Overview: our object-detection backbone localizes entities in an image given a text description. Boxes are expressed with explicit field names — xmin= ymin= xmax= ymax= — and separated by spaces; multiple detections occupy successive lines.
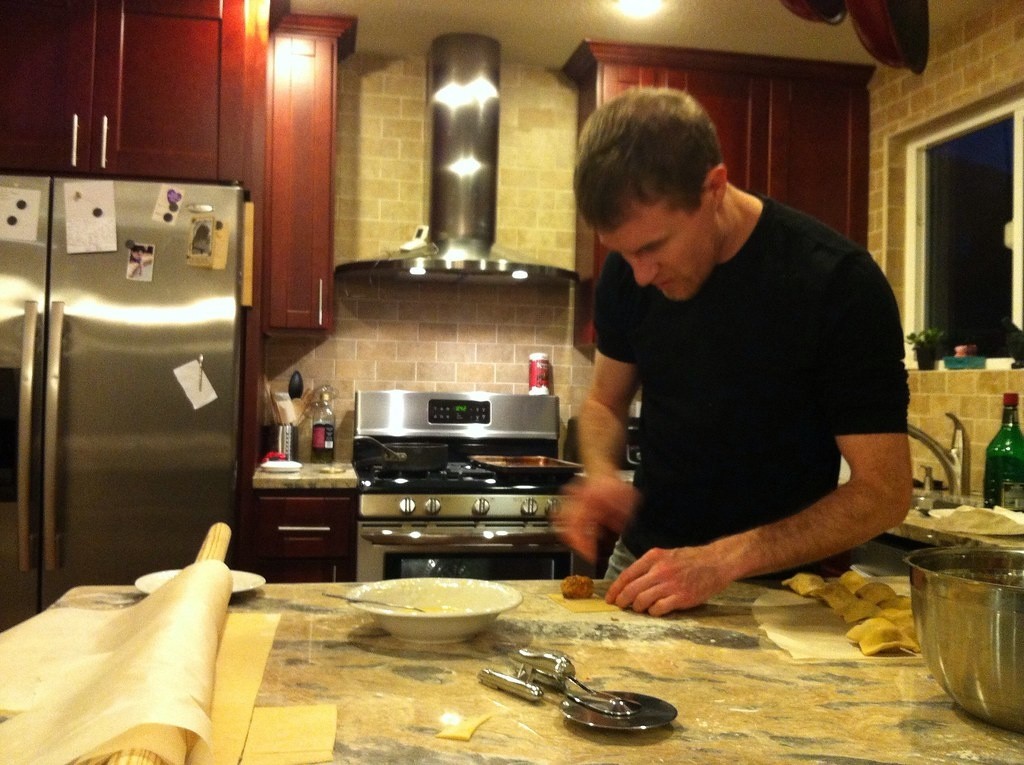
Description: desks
xmin=0 ymin=580 xmax=1024 ymax=765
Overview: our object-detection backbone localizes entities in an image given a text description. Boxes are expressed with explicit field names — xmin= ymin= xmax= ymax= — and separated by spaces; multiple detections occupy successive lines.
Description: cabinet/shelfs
xmin=262 ymin=29 xmax=337 ymax=338
xmin=560 ymin=40 xmax=877 ymax=350
xmin=251 ymin=490 xmax=359 ymax=584
xmin=0 ymin=0 xmax=271 ymax=188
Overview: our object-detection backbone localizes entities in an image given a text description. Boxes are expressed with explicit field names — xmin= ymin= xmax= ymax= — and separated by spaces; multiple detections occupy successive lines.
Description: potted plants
xmin=908 ymin=327 xmax=947 ymax=371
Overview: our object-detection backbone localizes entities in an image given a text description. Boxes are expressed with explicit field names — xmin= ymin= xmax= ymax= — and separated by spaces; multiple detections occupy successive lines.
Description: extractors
xmin=333 ymin=32 xmax=579 ymax=288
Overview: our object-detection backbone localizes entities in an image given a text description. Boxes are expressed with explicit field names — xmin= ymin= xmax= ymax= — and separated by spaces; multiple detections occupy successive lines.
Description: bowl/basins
xmin=347 ymin=577 xmax=523 ymax=644
xmin=903 ymin=546 xmax=1024 ymax=733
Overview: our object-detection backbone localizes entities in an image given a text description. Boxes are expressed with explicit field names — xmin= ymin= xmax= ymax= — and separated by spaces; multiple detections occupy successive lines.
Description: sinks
xmin=908 ymin=498 xmax=964 ymax=509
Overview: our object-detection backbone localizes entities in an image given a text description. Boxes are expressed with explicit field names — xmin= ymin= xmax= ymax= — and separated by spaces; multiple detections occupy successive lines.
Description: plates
xmin=260 ymin=460 xmax=302 ymax=472
xmin=929 ymin=507 xmax=1024 ymax=524
xmin=134 ymin=569 xmax=266 ymax=593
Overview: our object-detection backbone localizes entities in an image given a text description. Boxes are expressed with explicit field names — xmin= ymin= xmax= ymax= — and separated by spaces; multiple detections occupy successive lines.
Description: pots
xmin=353 ymin=435 xmax=448 ymax=471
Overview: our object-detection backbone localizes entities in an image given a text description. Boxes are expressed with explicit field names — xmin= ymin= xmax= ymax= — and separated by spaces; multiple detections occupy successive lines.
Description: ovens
xmin=356 ymin=521 xmax=596 ymax=583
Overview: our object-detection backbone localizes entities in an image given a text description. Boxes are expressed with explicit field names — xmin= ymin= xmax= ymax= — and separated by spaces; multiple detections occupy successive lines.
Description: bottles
xmin=311 ymin=392 xmax=335 ymax=463
xmin=982 ymin=392 xmax=1024 ymax=511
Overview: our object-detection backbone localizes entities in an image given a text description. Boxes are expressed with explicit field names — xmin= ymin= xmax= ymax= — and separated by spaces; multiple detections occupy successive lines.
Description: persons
xmin=558 ymin=88 xmax=912 ymax=616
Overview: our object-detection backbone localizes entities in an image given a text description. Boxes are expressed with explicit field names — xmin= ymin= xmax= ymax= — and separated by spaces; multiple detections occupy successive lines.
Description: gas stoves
xmin=356 ymin=390 xmax=587 ymax=523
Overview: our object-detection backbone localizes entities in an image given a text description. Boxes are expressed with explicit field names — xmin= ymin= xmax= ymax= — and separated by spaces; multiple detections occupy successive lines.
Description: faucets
xmin=907 ymin=411 xmax=971 ymax=494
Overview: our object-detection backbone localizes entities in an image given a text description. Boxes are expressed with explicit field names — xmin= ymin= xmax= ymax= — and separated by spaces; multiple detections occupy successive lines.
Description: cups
xmin=529 ymin=353 xmax=550 ymax=395
xmin=268 ymin=424 xmax=297 ymax=461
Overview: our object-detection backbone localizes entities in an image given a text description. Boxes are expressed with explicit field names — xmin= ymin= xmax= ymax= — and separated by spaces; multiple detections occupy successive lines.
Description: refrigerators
xmin=1 ymin=167 xmax=247 ymax=633
